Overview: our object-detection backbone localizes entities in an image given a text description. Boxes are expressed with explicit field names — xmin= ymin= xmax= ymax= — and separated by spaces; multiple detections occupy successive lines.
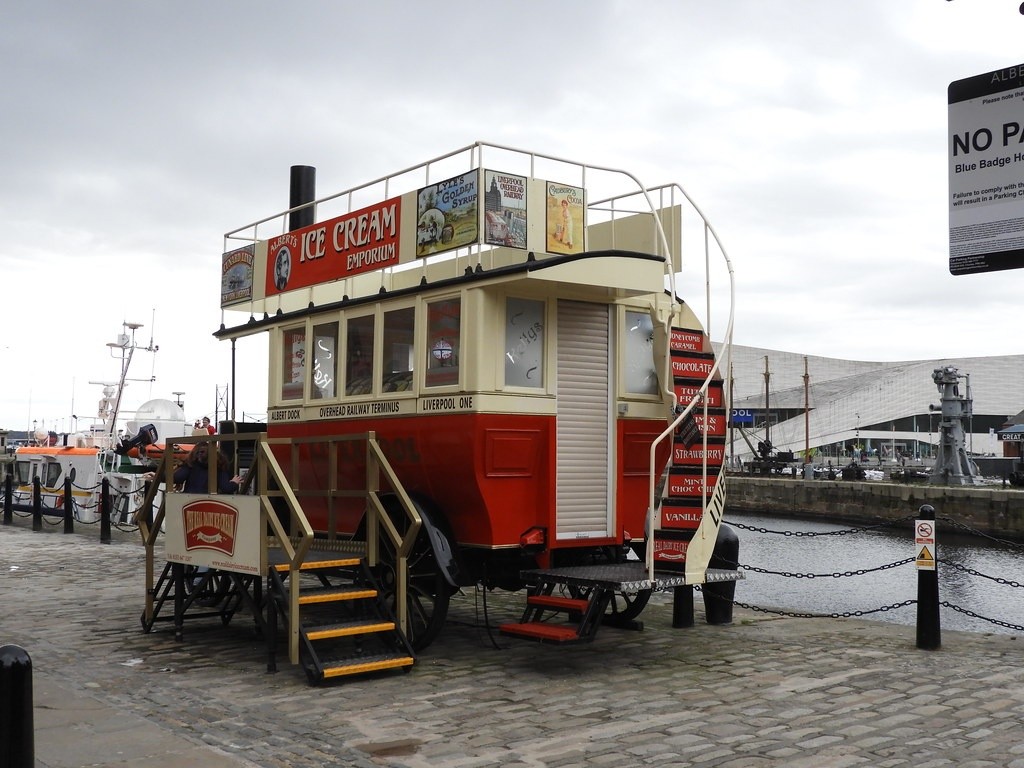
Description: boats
xmin=14 ymin=439 xmax=170 ymax=527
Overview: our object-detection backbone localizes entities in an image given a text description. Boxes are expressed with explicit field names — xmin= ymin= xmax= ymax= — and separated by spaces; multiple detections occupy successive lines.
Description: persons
xmin=142 ymin=443 xmax=244 ymax=494
xmin=201 ymin=417 xmax=215 ymax=435
